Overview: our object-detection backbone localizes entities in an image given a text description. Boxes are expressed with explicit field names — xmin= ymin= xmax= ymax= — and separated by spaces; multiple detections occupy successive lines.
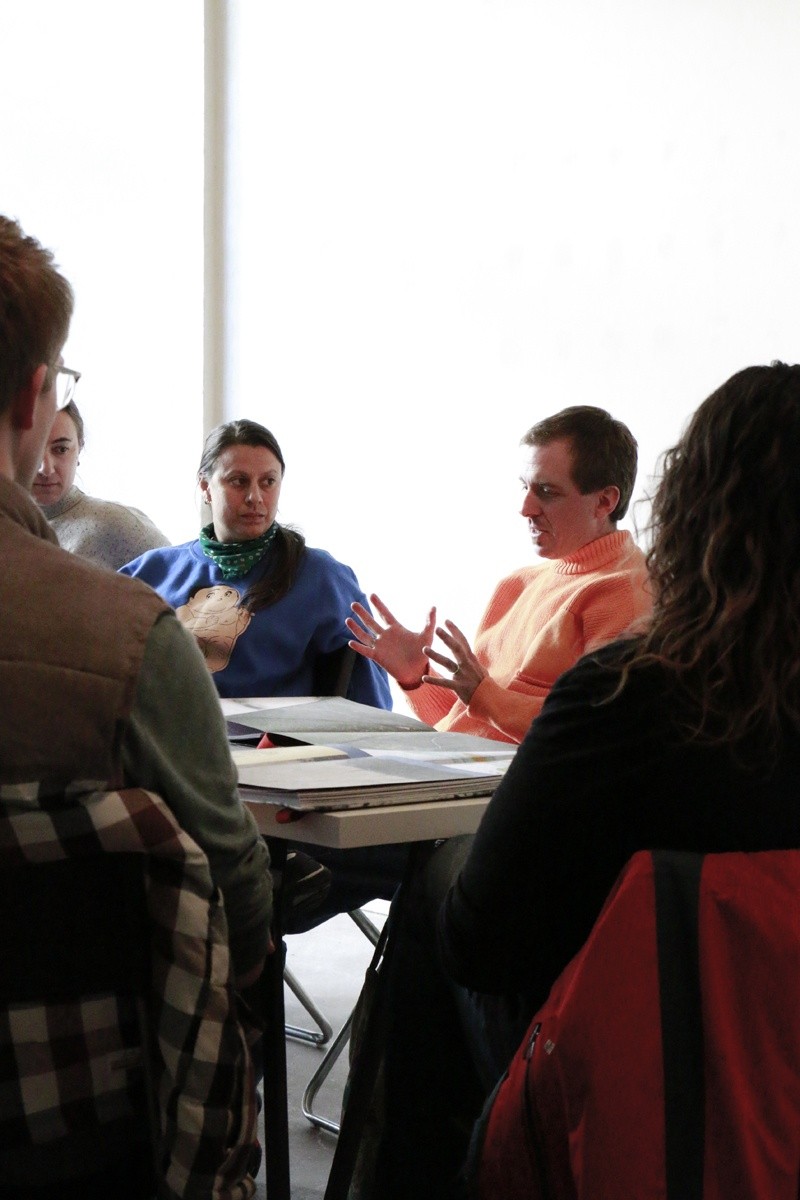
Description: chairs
xmin=468 ymin=848 xmax=800 ymax=1200
xmin=0 ymin=770 xmax=290 ymax=1200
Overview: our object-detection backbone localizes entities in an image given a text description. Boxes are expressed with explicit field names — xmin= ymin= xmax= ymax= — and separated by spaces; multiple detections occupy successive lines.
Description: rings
xmin=370 ymin=638 xmax=376 ymax=648
xmin=452 ymin=665 xmax=459 ymax=675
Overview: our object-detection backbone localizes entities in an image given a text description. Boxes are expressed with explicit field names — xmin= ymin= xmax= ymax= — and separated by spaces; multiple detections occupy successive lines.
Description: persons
xmin=0 ymin=213 xmax=387 ymax=1200
xmin=347 ymin=406 xmax=660 ymax=746
xmin=348 ymin=367 xmax=800 ymax=1200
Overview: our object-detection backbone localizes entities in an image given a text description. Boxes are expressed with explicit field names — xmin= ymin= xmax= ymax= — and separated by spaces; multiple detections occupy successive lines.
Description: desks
xmin=219 ymin=696 xmax=490 ymax=849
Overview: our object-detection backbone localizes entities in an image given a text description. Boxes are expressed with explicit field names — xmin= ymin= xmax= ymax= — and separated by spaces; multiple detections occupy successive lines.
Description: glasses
xmin=50 ymin=362 xmax=80 ymax=411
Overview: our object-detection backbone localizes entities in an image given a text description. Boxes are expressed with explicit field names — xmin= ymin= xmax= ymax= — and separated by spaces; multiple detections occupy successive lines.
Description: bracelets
xmin=398 ymin=662 xmax=430 ymax=690
